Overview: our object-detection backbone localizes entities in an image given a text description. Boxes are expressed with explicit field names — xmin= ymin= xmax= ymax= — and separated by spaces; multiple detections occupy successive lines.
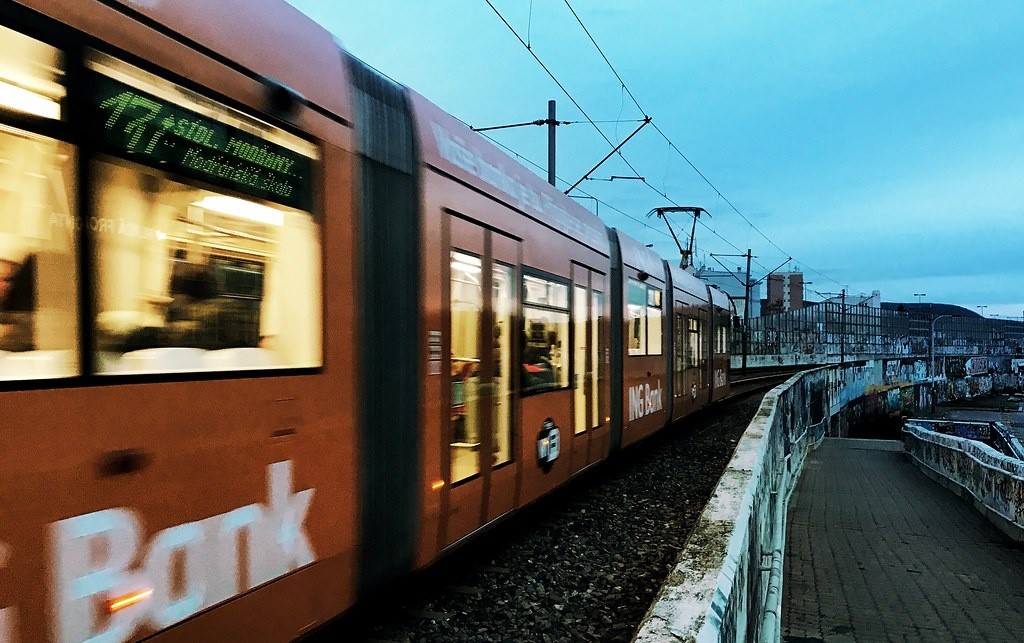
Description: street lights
xmin=930 ymin=315 xmax=953 ymax=388
xmin=914 ymin=293 xmax=926 ymax=305
xmin=977 ymin=306 xmax=987 ymax=317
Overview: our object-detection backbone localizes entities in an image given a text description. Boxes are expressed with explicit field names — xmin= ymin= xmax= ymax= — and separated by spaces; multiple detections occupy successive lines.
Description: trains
xmin=2 ymin=0 xmax=743 ymax=643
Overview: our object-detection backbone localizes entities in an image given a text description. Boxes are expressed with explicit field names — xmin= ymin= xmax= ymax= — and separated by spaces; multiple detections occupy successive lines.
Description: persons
xmin=0 ymin=259 xmax=34 ymax=351
xmin=97 ymin=264 xmax=259 ymax=351
